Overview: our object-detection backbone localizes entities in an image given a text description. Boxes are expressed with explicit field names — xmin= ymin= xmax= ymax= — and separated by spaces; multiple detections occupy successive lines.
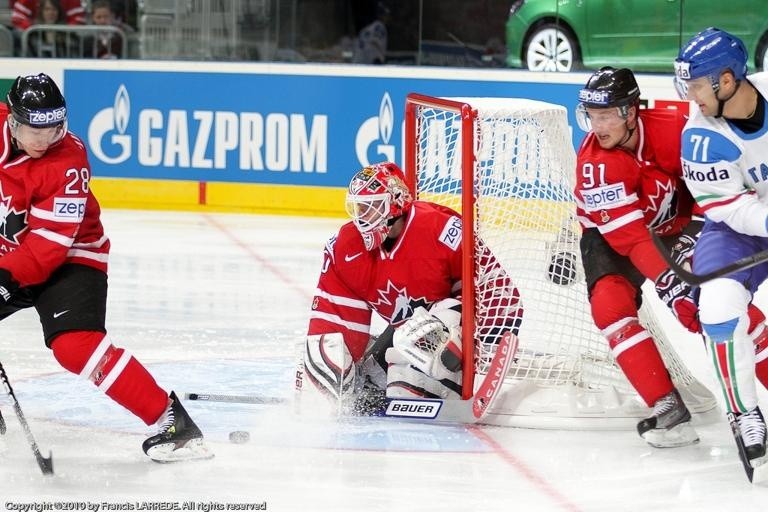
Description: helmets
xmin=345 ymin=163 xmax=414 ymax=251
xmin=576 ymin=67 xmax=640 ymax=132
xmin=673 ymin=27 xmax=748 ymax=100
xmin=7 ymin=73 xmax=67 ymax=149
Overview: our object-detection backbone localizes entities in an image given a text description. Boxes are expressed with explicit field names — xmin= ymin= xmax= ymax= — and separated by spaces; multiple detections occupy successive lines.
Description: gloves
xmin=655 ymin=269 xmax=703 ymax=334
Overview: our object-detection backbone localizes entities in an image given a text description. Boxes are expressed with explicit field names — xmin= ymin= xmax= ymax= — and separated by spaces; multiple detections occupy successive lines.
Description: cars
xmin=326 ymin=1 xmax=508 ymax=68
xmin=503 ymin=0 xmax=767 ymax=86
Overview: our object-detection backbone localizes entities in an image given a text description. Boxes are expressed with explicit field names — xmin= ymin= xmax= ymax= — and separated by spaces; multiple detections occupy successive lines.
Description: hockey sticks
xmin=649 ymin=226 xmax=766 ymax=286
xmin=183 ymin=331 xmax=519 ymax=422
xmin=1 ymin=362 xmax=54 ymax=475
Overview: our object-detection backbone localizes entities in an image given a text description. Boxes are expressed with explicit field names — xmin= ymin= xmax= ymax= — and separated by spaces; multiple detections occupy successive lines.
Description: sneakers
xmin=142 ymin=391 xmax=203 ymax=453
xmin=736 ymin=406 xmax=767 ymax=460
xmin=638 ymin=388 xmax=691 ymax=435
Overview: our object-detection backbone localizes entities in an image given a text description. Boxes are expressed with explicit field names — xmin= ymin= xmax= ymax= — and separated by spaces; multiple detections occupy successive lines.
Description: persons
xmin=574 ymin=66 xmax=767 ymax=441
xmin=1 ymin=72 xmax=215 ymax=456
xmin=304 ymin=162 xmax=523 ymax=417
xmin=0 ymin=0 xmax=514 ymax=66
xmin=673 ymin=27 xmax=767 ymax=482
